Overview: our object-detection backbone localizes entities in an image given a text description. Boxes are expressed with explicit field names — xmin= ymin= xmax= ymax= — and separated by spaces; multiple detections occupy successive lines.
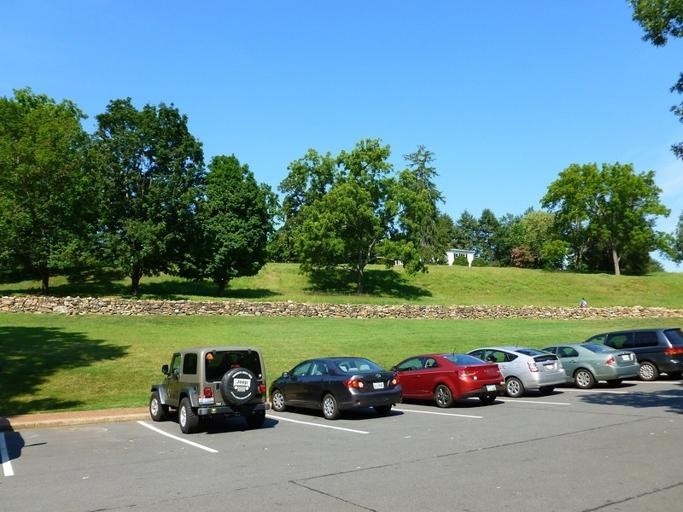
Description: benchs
xmin=340 ymin=365 xmax=370 ymax=373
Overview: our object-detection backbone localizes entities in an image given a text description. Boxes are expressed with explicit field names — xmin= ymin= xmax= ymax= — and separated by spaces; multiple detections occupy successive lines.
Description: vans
xmin=583 ymin=327 xmax=683 ymax=381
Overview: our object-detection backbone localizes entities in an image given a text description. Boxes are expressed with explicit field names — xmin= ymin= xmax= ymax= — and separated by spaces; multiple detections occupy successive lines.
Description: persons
xmin=580 ymin=297 xmax=588 ymax=307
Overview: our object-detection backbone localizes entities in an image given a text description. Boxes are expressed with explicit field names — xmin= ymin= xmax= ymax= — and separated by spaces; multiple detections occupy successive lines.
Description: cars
xmin=465 ymin=345 xmax=567 ymax=398
xmin=268 ymin=357 xmax=403 ymax=420
xmin=388 ymin=348 xmax=506 ymax=408
xmin=542 ymin=342 xmax=641 ymax=388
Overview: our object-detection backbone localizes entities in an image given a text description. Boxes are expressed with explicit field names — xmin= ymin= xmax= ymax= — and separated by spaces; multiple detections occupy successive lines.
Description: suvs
xmin=148 ymin=345 xmax=270 ymax=433
